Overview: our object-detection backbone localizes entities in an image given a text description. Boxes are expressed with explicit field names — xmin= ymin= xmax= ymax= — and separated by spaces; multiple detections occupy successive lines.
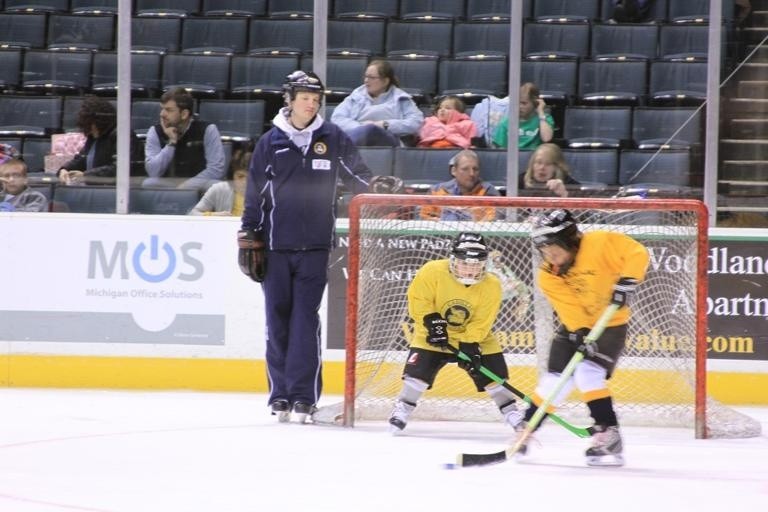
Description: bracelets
xmin=168 ymin=141 xmax=177 ymax=147
xmin=539 ymin=115 xmax=546 ymax=121
xmin=382 ymin=120 xmax=390 ymax=131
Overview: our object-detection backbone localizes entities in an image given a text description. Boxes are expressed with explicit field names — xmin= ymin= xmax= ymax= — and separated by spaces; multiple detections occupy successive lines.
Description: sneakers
xmin=272 ymin=401 xmax=292 ymax=411
xmin=295 ymin=401 xmax=317 ymax=414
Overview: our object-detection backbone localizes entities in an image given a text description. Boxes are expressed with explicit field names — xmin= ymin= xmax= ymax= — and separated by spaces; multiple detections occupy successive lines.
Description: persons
xmin=0 ymin=159 xmax=50 ymax=211
xmin=143 ymin=88 xmax=226 ymax=192
xmin=418 ymin=96 xmax=476 ymax=148
xmin=388 ymin=233 xmax=522 ymax=431
xmin=518 ymin=144 xmax=587 ymax=198
xmin=329 ymin=59 xmax=425 ymax=147
xmin=54 ymin=97 xmax=143 ymax=183
xmin=186 ymin=149 xmax=251 ymax=217
xmin=522 ymin=209 xmax=652 ymax=456
xmin=237 ymin=70 xmax=405 ymax=415
xmin=412 ymin=149 xmax=506 ymax=221
xmin=490 ymin=82 xmax=555 ymax=149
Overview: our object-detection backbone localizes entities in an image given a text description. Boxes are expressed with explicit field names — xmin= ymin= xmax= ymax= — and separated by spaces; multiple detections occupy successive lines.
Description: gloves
xmin=238 ymin=227 xmax=268 ymax=283
xmin=424 ymin=312 xmax=448 ymax=350
xmin=369 ymin=174 xmax=405 ymax=194
xmin=458 ymin=342 xmax=482 ymax=374
xmin=569 ymin=327 xmax=599 ymax=359
xmin=612 ymin=277 xmax=638 ymax=305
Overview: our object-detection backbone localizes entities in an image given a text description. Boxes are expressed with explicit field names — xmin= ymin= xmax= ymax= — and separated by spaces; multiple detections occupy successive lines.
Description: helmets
xmin=282 ymin=70 xmax=325 ymax=99
xmin=448 ymin=232 xmax=488 ymax=285
xmin=531 ymin=209 xmax=578 ymax=266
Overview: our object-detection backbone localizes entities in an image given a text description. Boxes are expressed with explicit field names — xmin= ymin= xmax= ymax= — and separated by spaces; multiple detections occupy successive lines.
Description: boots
xmin=389 ymin=398 xmax=417 ymax=430
xmin=501 ymin=399 xmax=536 ymax=453
xmin=586 ymin=425 xmax=623 ymax=456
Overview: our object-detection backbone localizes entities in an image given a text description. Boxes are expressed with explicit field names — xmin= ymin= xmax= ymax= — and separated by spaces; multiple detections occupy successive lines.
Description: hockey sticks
xmin=456 ymin=304 xmax=620 ymax=467
xmin=448 ymin=344 xmax=603 ymax=437
